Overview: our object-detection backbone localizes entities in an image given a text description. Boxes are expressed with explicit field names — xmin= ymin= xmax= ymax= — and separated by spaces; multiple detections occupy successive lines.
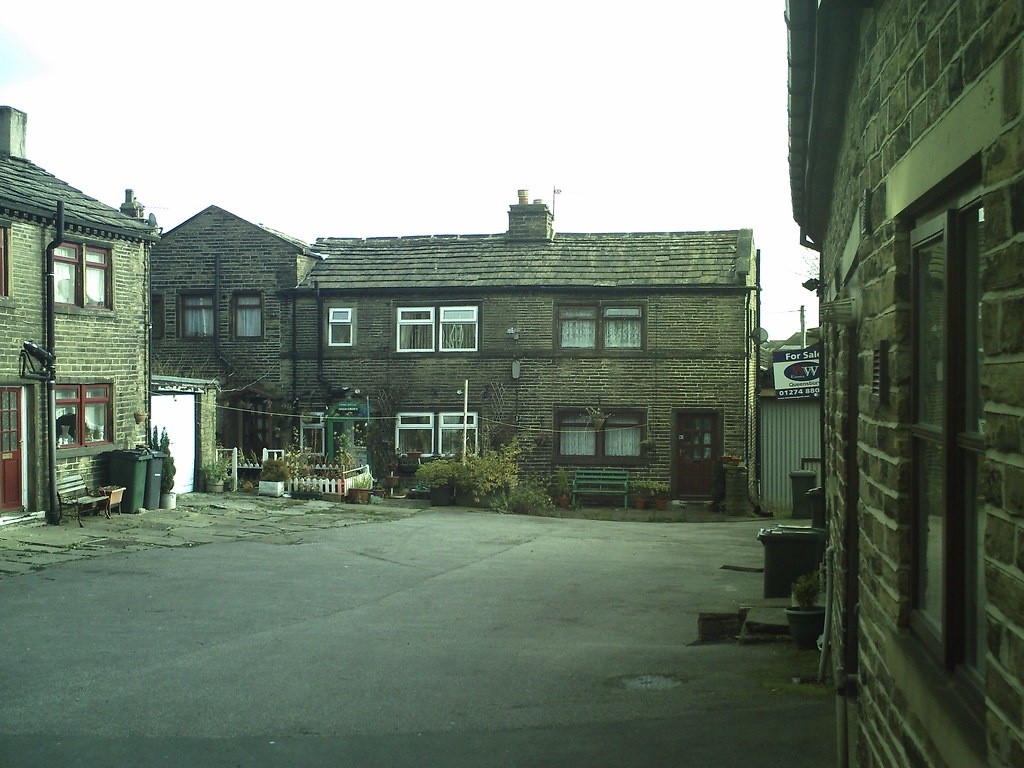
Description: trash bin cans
xmin=787 ymin=468 xmax=819 ymax=520
xmin=99 ymin=444 xmax=169 ymax=514
xmin=756 ymin=523 xmax=827 ymax=600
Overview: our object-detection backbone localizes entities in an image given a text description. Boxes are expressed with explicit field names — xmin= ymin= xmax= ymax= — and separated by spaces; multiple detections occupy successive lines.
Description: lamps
xmin=20 ymin=340 xmax=58 ymax=382
xmin=507 ymin=327 xmax=521 ymax=340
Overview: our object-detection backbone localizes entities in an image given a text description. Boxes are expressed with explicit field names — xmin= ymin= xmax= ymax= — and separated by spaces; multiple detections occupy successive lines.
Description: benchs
xmin=572 ymin=469 xmax=630 ymax=511
xmin=56 ymin=475 xmax=111 ymax=527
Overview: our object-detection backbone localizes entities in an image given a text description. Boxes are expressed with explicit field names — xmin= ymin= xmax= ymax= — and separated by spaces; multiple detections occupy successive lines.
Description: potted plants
xmin=732 ymin=455 xmax=741 ymax=466
xmin=555 ymin=467 xmax=571 ymax=509
xmin=257 ymin=457 xmax=291 ymax=497
xmin=415 ymin=448 xmax=520 ymax=508
xmin=160 ymin=427 xmax=176 ymax=510
xmin=631 ymin=478 xmax=671 ymax=511
xmin=199 ymin=457 xmax=231 ymax=492
xmin=785 ymin=569 xmax=829 ymax=653
xmin=348 ymin=460 xmax=399 ymax=503
xmin=720 ymin=453 xmax=733 ymax=464
xmin=578 ymin=405 xmax=612 ymax=430
xmin=94 ymin=480 xmax=126 ymax=519
